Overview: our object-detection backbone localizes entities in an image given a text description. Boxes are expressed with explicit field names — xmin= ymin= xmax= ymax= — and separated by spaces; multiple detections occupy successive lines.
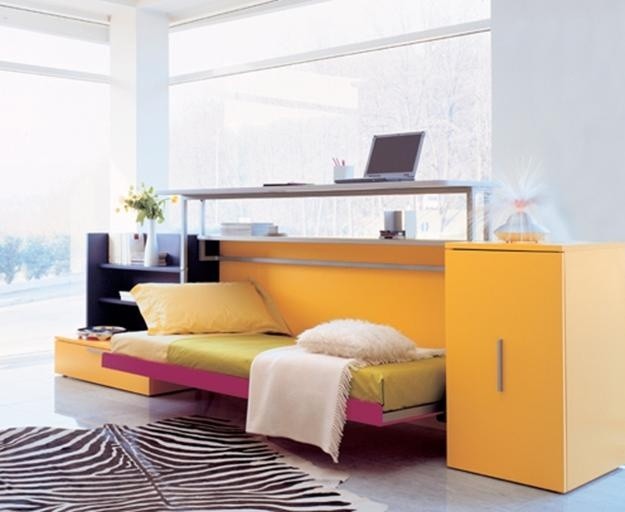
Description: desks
xmin=158 ymin=177 xmax=501 ymax=284
xmin=55 ymin=333 xmax=193 ymax=397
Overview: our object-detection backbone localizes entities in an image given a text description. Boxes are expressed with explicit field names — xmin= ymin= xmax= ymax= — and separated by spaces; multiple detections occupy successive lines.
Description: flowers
xmin=115 ymin=180 xmax=180 ymax=227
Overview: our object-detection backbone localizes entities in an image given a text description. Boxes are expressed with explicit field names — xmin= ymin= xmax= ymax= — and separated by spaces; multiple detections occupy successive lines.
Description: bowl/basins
xmin=118 ymin=291 xmax=135 ymax=302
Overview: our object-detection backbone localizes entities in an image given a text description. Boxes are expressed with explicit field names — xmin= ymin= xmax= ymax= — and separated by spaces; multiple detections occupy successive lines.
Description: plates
xmin=219 ymin=220 xmax=277 ymax=238
xmin=78 ymin=327 xmax=126 ymax=339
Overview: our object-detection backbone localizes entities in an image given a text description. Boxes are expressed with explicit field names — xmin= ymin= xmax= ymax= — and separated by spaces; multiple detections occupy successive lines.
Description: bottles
xmin=130 ymin=233 xmax=143 ymax=264
xmin=493 ymin=212 xmax=548 ymax=243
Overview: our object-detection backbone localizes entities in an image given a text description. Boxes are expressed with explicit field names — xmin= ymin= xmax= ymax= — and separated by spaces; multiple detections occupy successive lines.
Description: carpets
xmin=0 ymin=413 xmax=389 ymax=512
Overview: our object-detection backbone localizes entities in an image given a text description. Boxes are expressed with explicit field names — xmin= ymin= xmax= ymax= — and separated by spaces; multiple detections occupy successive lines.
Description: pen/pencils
xmin=333 ymin=158 xmax=346 ymax=167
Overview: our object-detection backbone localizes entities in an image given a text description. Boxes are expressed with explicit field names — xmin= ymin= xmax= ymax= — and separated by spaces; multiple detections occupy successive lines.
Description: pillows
xmin=131 ymin=280 xmax=292 ymax=336
xmin=294 ymin=317 xmax=417 ymax=364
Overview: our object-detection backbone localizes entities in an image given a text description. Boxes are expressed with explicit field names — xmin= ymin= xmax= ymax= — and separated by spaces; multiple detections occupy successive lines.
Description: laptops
xmin=334 ymin=131 xmax=425 ymax=182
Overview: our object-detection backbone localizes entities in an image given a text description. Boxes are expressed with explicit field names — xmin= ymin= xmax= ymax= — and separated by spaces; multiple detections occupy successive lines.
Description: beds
xmin=101 ymin=331 xmax=445 ymax=427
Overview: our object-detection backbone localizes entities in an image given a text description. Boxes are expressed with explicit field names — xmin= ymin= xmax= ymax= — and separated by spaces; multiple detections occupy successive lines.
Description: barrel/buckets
xmin=77 ymin=321 xmax=128 ymax=345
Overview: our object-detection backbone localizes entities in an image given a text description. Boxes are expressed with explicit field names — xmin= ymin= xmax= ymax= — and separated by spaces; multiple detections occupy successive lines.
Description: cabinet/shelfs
xmin=99 ymin=262 xmax=180 ymax=308
xmin=444 ymin=242 xmax=625 ymax=495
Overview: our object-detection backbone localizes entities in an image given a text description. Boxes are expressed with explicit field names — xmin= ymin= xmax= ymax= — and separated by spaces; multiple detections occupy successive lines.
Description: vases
xmin=143 ymin=220 xmax=159 ymax=267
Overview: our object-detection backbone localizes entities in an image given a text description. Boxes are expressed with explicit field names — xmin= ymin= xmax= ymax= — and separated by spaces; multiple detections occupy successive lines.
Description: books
xmin=221 ymin=222 xmax=279 ymax=237
xmin=108 ymin=232 xmax=168 ymax=266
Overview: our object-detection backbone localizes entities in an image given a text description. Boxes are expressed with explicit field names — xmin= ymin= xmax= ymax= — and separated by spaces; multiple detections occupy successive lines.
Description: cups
xmin=332 ymin=166 xmax=353 ymax=183
